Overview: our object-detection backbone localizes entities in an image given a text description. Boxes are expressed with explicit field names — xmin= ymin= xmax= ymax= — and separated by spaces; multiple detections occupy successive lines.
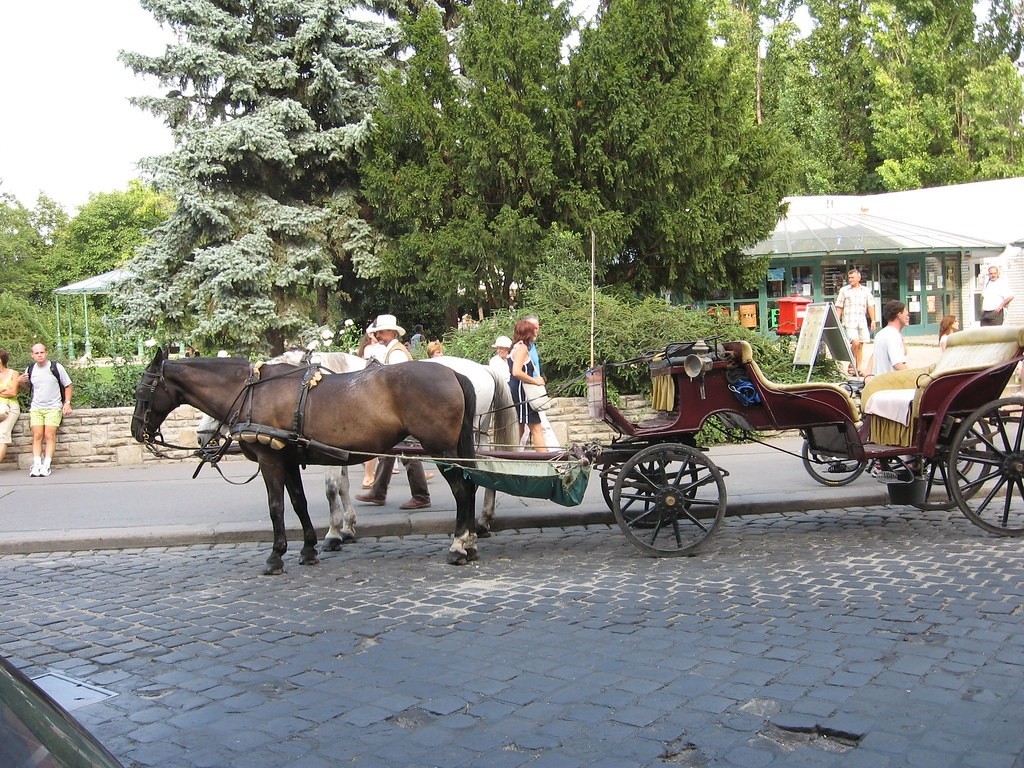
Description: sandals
xmin=858 ymin=370 xmax=865 ymax=378
xmin=849 ymin=363 xmax=855 ymax=375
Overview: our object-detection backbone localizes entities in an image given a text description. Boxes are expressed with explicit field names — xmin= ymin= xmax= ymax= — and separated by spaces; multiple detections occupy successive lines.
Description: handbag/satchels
xmin=0 ymin=402 xmax=10 ymax=421
xmin=513 ymin=344 xmax=551 ymax=411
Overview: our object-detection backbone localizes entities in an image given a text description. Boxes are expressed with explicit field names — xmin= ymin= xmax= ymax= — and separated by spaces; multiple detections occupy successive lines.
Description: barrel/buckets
xmin=887 ymin=467 xmax=927 ymax=505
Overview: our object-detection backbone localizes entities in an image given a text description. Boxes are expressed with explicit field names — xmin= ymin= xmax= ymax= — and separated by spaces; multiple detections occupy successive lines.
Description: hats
xmin=491 ymin=336 xmax=513 ymax=349
xmin=368 ymin=314 xmax=406 ymax=337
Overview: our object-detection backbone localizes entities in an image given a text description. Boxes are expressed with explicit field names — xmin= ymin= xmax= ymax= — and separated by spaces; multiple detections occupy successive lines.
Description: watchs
xmin=64 ymin=400 xmax=71 ymax=404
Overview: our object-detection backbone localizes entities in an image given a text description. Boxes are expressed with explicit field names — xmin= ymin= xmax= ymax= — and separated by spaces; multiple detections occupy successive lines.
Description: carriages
xmin=130 ymin=325 xmax=1023 ymax=576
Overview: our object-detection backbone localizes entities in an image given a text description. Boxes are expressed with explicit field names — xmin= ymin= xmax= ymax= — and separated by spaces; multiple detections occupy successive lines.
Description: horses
xmin=196 ymin=350 xmax=520 ymax=551
xmin=131 ymin=347 xmax=480 ymax=576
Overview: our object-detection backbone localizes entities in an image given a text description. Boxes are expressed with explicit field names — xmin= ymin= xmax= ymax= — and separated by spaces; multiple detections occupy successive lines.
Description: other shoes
xmin=362 ymin=482 xmax=375 ymax=490
xmin=391 ymin=469 xmax=400 ymax=474
xmin=425 ymin=472 xmax=434 ymax=479
xmin=872 ymin=467 xmax=883 ymax=477
xmin=399 ymin=495 xmax=431 ymax=510
xmin=355 ymin=491 xmax=386 ymax=505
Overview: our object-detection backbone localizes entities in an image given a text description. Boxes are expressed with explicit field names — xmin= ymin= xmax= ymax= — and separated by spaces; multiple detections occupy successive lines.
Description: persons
xmin=0 ymin=350 xmax=20 ymax=462
xmin=980 ymin=266 xmax=1013 ymax=325
xmin=520 ymin=316 xmax=561 ymax=451
xmin=865 ymin=300 xmax=909 ymax=384
xmin=488 ymin=336 xmax=511 ymax=449
xmin=938 ymin=314 xmax=959 ymax=351
xmin=18 ymin=344 xmax=73 ymax=477
xmin=355 ymin=314 xmax=435 ymax=509
xmin=835 ymin=268 xmax=876 ymax=377
xmin=507 ymin=321 xmax=547 ymax=453
xmin=427 ymin=341 xmax=443 ymax=358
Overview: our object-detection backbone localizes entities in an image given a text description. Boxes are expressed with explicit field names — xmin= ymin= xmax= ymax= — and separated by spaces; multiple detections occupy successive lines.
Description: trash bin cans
xmin=775 ymin=297 xmax=813 ymax=336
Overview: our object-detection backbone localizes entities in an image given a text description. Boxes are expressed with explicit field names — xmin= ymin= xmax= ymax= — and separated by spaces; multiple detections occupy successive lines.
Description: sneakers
xmin=30 ymin=464 xmax=41 ymax=477
xmin=41 ymin=462 xmax=51 ymax=478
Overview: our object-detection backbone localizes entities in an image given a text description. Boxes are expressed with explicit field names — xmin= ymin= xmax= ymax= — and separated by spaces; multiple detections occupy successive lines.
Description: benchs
xmin=645 ymin=335 xmax=727 ymax=377
xmin=723 ymin=341 xmax=860 ymax=422
xmin=860 ymin=325 xmax=1024 ymax=413
